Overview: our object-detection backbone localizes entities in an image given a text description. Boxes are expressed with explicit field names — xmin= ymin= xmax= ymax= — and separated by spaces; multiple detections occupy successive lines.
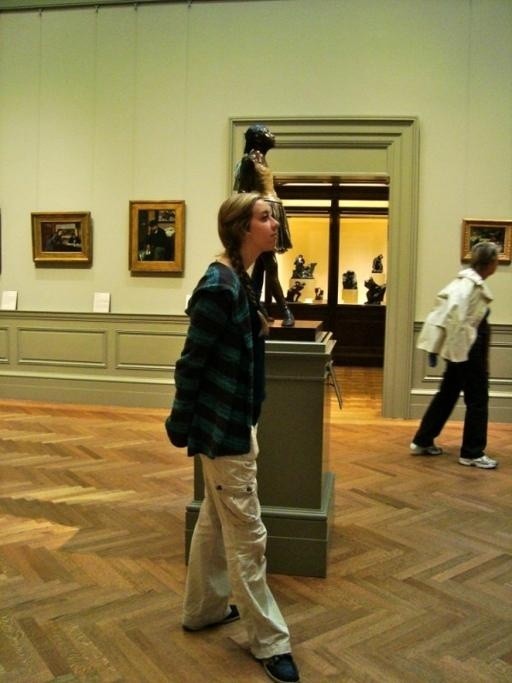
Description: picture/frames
xmin=30 ymin=210 xmax=90 ymax=265
xmin=127 ymin=198 xmax=186 ymax=276
xmin=461 ymin=218 xmax=512 ymax=266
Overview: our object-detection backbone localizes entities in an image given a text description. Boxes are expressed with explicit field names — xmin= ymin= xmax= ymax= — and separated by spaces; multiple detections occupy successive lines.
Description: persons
xmin=408 ymin=240 xmax=499 ymax=469
xmin=232 ymin=124 xmax=294 ymax=328
xmin=45 ymin=229 xmax=65 ymax=252
xmin=165 ymin=192 xmax=300 ymax=683
xmin=144 ymin=219 xmax=170 ymax=261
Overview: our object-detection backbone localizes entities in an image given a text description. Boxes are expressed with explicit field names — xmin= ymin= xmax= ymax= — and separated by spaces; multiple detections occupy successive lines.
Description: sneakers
xmin=183 ymin=604 xmax=239 ymax=632
xmin=261 ymin=654 xmax=300 ymax=683
xmin=458 ymin=455 xmax=498 ymax=469
xmin=409 ymin=442 xmax=442 ymax=455
